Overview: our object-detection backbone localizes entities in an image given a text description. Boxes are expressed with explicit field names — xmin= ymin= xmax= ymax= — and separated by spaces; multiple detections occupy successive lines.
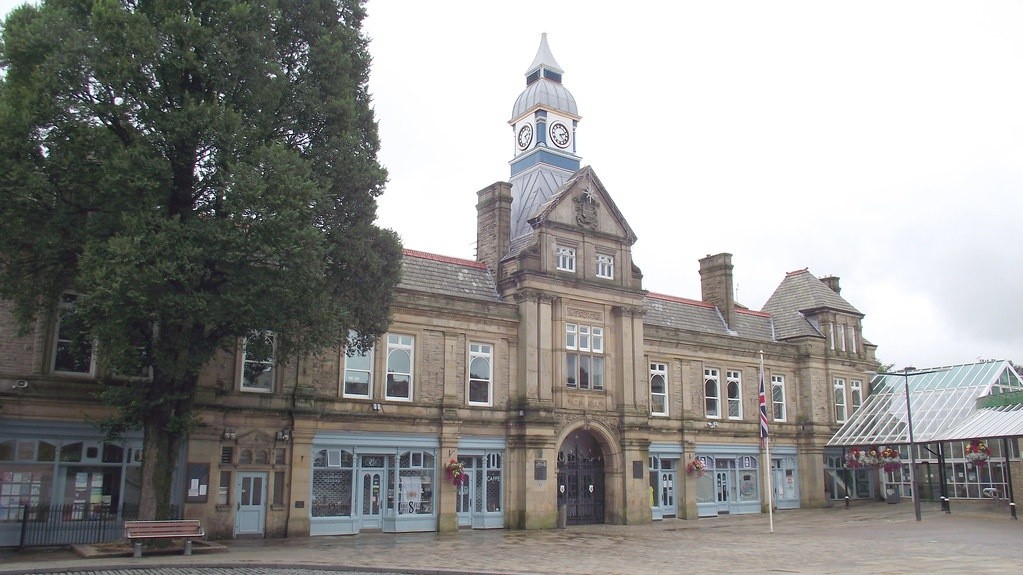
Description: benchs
xmin=123 ymin=520 xmax=206 ymax=557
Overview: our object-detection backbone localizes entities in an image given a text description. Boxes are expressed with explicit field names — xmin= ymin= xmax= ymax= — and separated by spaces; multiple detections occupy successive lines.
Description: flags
xmin=758 ymin=378 xmax=768 ymax=441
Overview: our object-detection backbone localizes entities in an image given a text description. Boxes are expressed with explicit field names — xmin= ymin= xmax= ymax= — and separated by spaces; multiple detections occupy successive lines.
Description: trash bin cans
xmin=886 ymin=483 xmax=900 ymax=504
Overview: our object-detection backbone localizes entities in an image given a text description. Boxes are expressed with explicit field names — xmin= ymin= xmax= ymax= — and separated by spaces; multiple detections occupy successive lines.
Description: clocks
xmin=550 ymin=120 xmax=572 ymax=148
xmin=517 ymin=123 xmax=533 ymax=150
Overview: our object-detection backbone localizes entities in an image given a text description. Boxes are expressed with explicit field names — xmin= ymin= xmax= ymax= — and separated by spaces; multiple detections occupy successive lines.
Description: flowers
xmin=446 ymin=459 xmax=466 ymax=487
xmin=966 ymin=437 xmax=993 ymax=465
xmin=844 ymin=447 xmax=902 ymax=471
xmin=687 ymin=458 xmax=705 ymax=478
